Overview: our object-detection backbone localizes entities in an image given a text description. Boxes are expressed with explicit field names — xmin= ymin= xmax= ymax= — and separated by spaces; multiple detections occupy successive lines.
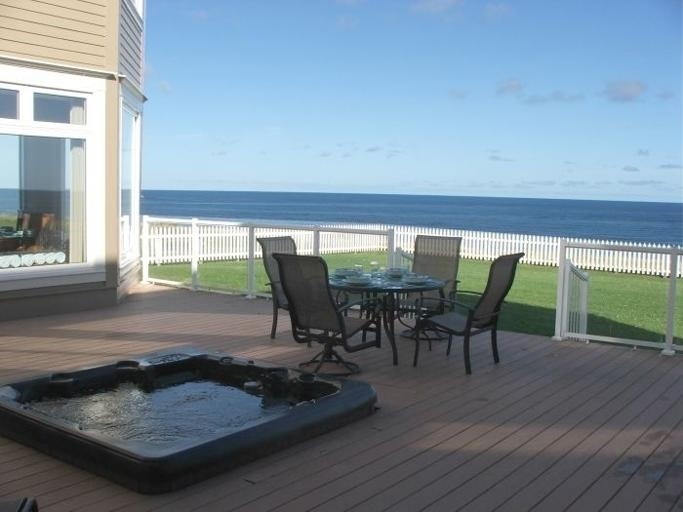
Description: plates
xmin=401 ymin=279 xmax=432 ymax=285
xmin=342 ymin=279 xmax=371 ymax=286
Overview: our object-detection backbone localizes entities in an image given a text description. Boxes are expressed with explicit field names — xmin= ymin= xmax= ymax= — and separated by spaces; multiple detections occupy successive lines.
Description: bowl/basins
xmin=346 ymin=275 xmax=369 ymax=282
xmin=407 ymin=275 xmax=428 ymax=281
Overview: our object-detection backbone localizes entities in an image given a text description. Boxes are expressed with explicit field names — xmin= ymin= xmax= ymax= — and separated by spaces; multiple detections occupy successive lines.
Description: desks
xmin=329 ymin=269 xmax=447 ymax=366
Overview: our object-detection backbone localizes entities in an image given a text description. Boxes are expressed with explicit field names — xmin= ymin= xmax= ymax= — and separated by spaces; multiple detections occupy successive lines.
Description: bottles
xmin=355 ymin=263 xmax=362 ymax=276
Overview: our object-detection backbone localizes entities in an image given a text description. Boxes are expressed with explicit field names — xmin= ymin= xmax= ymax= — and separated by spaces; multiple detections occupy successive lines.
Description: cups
xmin=369 ymin=261 xmax=383 ymax=279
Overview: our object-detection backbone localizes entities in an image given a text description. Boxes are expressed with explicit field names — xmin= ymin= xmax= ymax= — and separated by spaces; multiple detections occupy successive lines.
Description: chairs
xmin=256 ymin=235 xmax=342 ymax=345
xmin=272 ymin=252 xmax=373 ymax=377
xmin=413 ymin=252 xmax=526 ymax=374
xmin=395 ymin=234 xmax=462 ymax=350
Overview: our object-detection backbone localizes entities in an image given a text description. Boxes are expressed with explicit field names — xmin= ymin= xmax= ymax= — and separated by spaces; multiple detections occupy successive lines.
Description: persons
xmin=14 ymin=212 xmax=53 ymax=252
